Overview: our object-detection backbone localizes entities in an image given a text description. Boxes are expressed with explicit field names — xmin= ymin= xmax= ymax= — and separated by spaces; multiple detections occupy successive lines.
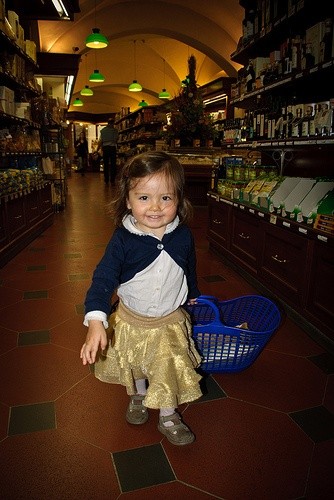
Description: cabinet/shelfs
xmin=0 ymin=0 xmax=57 ymax=269
xmin=114 ymin=106 xmax=158 ymax=144
xmin=184 ymin=1 xmax=334 ymax=372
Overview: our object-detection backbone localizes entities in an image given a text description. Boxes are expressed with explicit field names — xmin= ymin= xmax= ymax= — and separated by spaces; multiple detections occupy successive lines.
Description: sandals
xmin=127 ymin=395 xmax=148 ymax=425
xmin=157 ymin=413 xmax=195 ymax=445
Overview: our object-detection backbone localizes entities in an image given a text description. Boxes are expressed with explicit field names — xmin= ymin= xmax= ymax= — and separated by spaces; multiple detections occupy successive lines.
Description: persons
xmin=97 ymin=119 xmax=120 ymax=184
xmin=74 ymin=132 xmax=89 ymax=176
xmin=80 ymin=152 xmax=201 ymax=446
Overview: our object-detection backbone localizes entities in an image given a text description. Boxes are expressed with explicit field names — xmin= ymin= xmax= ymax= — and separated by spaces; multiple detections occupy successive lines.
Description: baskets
xmin=183 ymin=295 xmax=280 ymax=373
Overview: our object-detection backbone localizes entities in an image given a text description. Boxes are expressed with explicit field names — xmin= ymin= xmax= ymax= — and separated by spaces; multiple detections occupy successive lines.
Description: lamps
xmin=159 ymin=58 xmax=172 ymax=98
xmin=72 ymin=97 xmax=84 ymax=107
xmin=180 ymin=49 xmax=190 ymax=86
xmin=86 ymin=0 xmax=109 ymax=48
xmin=129 ymin=40 xmax=142 ymax=92
xmin=89 ymin=49 xmax=105 ymax=82
xmin=79 ymin=53 xmax=94 ymax=96
xmin=138 ymin=99 xmax=147 ymax=107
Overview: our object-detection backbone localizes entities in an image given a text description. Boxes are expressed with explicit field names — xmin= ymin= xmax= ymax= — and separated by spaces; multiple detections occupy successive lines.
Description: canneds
xmin=217 ymin=163 xmax=278 ymax=200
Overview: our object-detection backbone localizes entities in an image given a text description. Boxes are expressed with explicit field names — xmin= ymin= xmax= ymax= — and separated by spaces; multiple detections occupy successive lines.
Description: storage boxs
xmin=0 ymin=9 xmax=36 ymax=120
xmin=246 ymin=22 xmax=330 ymax=85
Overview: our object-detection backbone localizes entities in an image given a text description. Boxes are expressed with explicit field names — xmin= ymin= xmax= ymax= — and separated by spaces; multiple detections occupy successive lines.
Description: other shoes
xmin=104 ymin=179 xmax=109 ymax=183
xmin=111 ymin=179 xmax=115 ymax=182
